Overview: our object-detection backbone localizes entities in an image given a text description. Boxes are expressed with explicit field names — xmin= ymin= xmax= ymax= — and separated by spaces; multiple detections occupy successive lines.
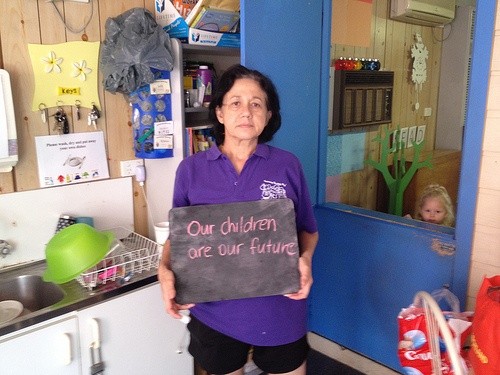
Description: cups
xmin=77 ymin=217 xmax=93 ymax=227
xmin=55 ymin=216 xmax=77 ymax=233
xmin=154 ymin=222 xmax=170 ymax=254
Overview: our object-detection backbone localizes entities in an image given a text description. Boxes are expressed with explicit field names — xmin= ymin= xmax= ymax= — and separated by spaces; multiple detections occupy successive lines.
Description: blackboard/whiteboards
xmin=167 ymin=198 xmax=300 ymax=305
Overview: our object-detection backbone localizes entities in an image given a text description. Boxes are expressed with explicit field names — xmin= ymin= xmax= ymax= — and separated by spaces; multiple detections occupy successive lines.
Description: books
xmin=173 ymin=0 xmax=240 ymax=33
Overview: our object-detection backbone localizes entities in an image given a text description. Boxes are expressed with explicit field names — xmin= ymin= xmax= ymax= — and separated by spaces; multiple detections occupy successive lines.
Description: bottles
xmin=197 ymin=65 xmax=212 ymax=102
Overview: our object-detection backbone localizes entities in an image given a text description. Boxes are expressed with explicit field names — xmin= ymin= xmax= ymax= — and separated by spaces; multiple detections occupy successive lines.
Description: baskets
xmin=75 ymin=224 xmax=166 ymax=290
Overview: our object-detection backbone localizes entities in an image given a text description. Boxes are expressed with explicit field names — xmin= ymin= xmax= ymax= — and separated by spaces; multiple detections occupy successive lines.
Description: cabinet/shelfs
xmin=144 ymin=38 xmax=241 ymax=243
xmin=0 ymin=234 xmax=195 ymax=375
xmin=392 ymin=148 xmax=460 ymax=228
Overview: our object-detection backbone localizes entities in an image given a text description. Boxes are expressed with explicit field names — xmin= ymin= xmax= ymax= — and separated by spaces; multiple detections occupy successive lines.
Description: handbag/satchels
xmin=468 ymin=276 xmax=500 ymax=375
xmin=398 ymin=286 xmax=472 ymax=374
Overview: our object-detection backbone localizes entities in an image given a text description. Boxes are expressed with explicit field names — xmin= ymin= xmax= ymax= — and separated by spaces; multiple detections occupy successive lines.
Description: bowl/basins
xmin=43 ymin=223 xmax=114 ymax=285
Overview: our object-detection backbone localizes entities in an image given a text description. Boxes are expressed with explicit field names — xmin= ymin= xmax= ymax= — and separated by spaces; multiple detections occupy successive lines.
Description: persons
xmin=158 ymin=64 xmax=316 ymax=375
xmin=402 ymin=183 xmax=455 ymax=226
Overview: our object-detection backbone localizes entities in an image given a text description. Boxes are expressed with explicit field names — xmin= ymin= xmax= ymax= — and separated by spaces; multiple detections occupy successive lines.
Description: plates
xmin=0 ymin=300 xmax=23 ymax=324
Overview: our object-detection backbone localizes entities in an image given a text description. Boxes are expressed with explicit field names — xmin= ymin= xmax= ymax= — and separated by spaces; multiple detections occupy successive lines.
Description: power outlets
xmin=120 ymin=158 xmax=144 ymax=177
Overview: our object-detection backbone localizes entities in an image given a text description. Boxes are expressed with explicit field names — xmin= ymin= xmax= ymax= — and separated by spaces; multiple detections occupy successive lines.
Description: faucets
xmin=0 ymin=240 xmax=12 ymax=258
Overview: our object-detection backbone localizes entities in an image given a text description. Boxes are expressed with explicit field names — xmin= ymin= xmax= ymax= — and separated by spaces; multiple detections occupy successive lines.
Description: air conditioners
xmin=389 ymin=0 xmax=455 ymax=28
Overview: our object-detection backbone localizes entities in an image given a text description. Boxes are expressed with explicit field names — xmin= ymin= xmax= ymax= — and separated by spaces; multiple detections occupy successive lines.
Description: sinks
xmin=0 ymin=271 xmax=68 ymax=327
